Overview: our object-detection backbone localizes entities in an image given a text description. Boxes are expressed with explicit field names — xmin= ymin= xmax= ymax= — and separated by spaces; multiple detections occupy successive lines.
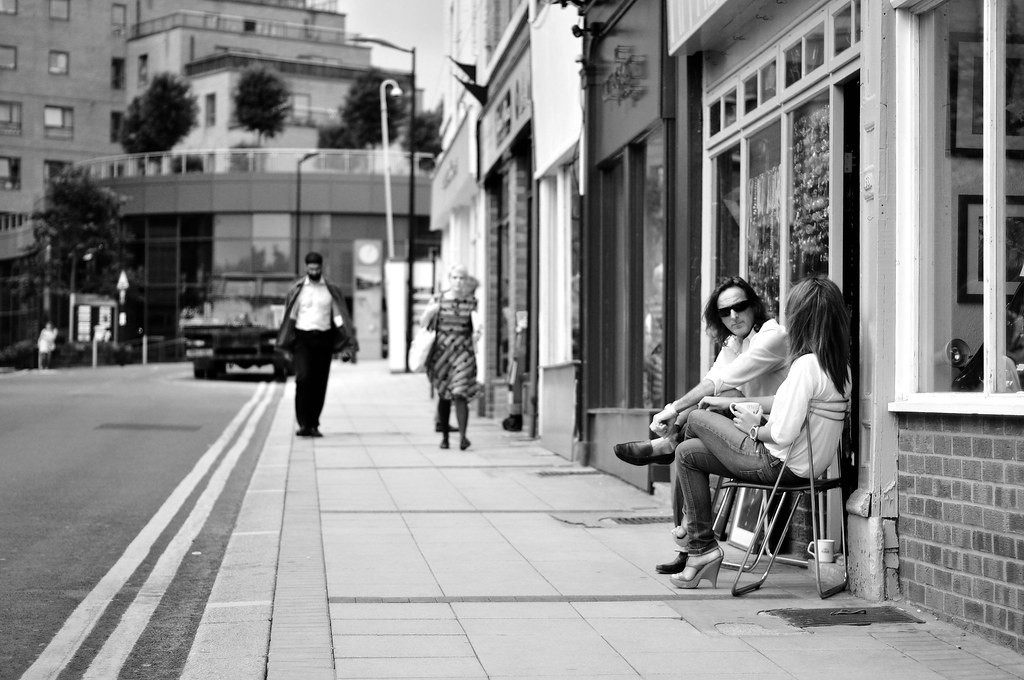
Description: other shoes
xmin=459 ymin=438 xmax=470 ymax=450
xmin=439 ymin=435 xmax=450 ymax=448
xmin=296 ymin=426 xmax=323 ymax=437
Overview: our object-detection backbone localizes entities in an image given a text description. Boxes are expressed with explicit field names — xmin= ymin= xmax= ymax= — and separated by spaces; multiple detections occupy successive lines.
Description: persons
xmin=613 ymin=277 xmax=790 ymax=573
xmin=419 ymin=263 xmax=481 ymax=450
xmin=643 ymin=264 xmax=664 ymax=410
xmin=38 ymin=320 xmax=59 ymax=370
xmin=668 ymin=277 xmax=852 ymax=589
xmin=275 ymin=251 xmax=356 ymax=437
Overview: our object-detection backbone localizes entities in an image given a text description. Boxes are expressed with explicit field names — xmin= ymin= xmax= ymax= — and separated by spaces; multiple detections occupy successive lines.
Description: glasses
xmin=713 ymin=300 xmax=749 ymax=319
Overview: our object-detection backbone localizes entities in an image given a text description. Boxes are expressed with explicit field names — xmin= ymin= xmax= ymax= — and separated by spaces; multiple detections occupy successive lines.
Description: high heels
xmin=670 ymin=526 xmax=691 ymax=546
xmin=670 ymin=551 xmax=724 ymax=590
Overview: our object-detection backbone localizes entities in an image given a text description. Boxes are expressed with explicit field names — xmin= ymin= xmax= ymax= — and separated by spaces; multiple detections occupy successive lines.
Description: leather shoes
xmin=656 ymin=551 xmax=688 ymax=574
xmin=613 ymin=440 xmax=675 ymax=467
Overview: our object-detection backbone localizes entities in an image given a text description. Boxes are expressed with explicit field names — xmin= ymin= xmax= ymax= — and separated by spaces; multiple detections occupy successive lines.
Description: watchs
xmin=749 ymin=424 xmax=762 ymax=441
xmin=665 ymin=404 xmax=679 ymax=417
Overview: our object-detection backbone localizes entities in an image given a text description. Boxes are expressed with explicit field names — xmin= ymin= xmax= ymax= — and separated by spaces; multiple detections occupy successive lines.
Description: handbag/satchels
xmin=408 ymin=295 xmax=441 ymax=371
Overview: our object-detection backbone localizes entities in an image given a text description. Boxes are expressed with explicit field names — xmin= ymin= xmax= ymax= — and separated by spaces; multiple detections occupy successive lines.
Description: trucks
xmin=178 ymin=273 xmax=301 ymax=383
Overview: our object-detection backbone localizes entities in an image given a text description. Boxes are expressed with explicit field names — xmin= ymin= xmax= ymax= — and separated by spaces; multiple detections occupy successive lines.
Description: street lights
xmin=295 ymin=151 xmax=321 ymax=274
xmin=345 ymin=37 xmax=418 ymax=374
xmin=379 ymin=78 xmax=403 ymax=359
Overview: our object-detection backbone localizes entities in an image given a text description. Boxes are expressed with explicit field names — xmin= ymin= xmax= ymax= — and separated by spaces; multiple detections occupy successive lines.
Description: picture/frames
xmin=949 ymin=33 xmax=1024 ymax=156
xmin=956 ymin=193 xmax=1024 ymax=304
xmin=726 ymin=486 xmax=772 ymax=554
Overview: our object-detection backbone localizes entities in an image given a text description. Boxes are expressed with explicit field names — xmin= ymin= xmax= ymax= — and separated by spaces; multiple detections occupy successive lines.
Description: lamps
xmin=932 ymin=338 xmax=969 ymax=371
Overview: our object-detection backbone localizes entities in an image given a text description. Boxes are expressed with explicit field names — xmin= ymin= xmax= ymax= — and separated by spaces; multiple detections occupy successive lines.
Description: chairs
xmin=720 ymin=399 xmax=851 ymax=599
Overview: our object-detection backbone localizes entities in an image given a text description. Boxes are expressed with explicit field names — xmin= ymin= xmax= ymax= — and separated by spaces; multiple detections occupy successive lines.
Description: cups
xmin=729 ymin=402 xmax=759 ymax=417
xmin=807 ymin=540 xmax=835 ymax=562
